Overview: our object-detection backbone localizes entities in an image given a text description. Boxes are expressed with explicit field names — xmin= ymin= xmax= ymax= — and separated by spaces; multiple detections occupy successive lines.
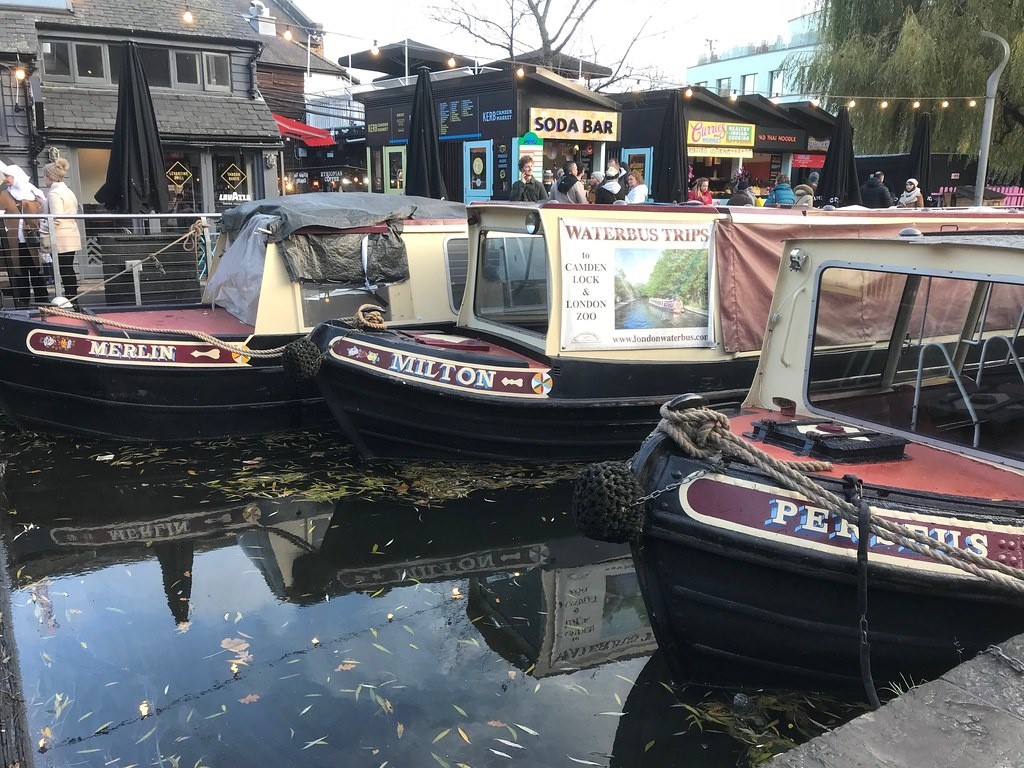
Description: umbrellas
xmin=813 ymin=106 xmax=862 ymax=208
xmin=338 ymin=39 xmax=479 ymax=86
xmin=405 ymin=66 xmax=450 ymax=201
xmin=478 ymin=47 xmax=613 ymax=79
xmin=898 ymin=112 xmax=933 ymax=207
xmin=94 ymin=37 xmax=175 ymax=234
xmin=646 ymin=90 xmax=689 ymax=203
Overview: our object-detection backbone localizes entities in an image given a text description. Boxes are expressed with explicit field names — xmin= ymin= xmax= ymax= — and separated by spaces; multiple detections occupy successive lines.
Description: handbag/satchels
xmin=27 ymin=229 xmax=40 ymax=246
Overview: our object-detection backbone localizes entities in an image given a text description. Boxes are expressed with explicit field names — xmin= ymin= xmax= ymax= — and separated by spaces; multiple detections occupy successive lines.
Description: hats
xmin=605 ymin=167 xmax=619 ymax=180
xmin=907 ymin=178 xmax=918 ymax=187
xmin=738 ymin=180 xmax=749 ymax=190
xmin=43 ymin=158 xmax=69 ymax=182
xmin=591 ymin=171 xmax=604 ymax=181
xmin=809 ymin=172 xmax=819 ymax=182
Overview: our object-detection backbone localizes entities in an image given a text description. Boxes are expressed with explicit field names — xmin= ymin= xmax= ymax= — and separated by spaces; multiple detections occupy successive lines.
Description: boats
xmin=648 ymin=296 xmax=685 ymax=315
xmin=0 ymin=191 xmax=554 ymax=452
xmin=0 ymin=420 xmax=657 ymax=682
xmin=568 ymin=229 xmax=1024 ymax=704
xmin=281 ymin=200 xmax=1024 ymax=463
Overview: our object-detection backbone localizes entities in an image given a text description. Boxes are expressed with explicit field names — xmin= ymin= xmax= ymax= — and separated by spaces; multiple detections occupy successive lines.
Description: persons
xmin=687 ymin=172 xmax=820 ymax=209
xmin=0 ymin=157 xmax=83 ymax=310
xmin=856 ymin=171 xmax=924 ymax=208
xmin=544 ymin=157 xmax=648 ymax=204
xmin=509 ymin=156 xmax=549 ymax=201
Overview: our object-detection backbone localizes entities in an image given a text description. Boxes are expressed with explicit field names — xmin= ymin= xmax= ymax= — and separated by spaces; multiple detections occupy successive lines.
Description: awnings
xmin=272 ymin=112 xmax=337 ymax=146
